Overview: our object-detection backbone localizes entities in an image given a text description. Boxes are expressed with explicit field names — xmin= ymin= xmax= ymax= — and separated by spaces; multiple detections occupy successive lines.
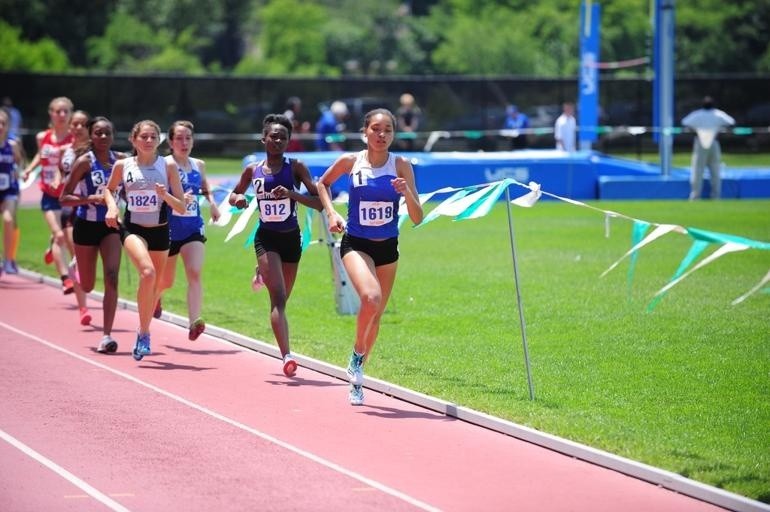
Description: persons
xmin=553 ymin=102 xmax=577 ymax=151
xmin=58 ymin=116 xmax=130 ymax=354
xmin=316 ymin=102 xmax=349 ymax=150
xmin=504 ymin=105 xmax=528 ymax=151
xmin=394 ymin=93 xmax=423 ymax=151
xmin=228 ymin=114 xmax=324 ymax=377
xmin=103 ymin=119 xmax=187 ymax=361
xmin=153 ymin=120 xmax=220 ymax=341
xmin=680 ymin=96 xmax=736 ymax=200
xmin=282 ymin=96 xmax=310 ymax=151
xmin=0 ymin=96 xmax=93 ymax=326
xmin=315 ymin=109 xmax=424 ymax=407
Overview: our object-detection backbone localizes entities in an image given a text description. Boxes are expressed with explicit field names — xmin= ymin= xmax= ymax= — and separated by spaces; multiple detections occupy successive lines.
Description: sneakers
xmin=153 ymin=299 xmax=162 ymax=318
xmin=189 ymin=317 xmax=205 ymax=340
xmin=97 ymin=336 xmax=117 ymax=354
xmin=45 ymin=235 xmax=55 ymax=263
xmin=3 ymin=259 xmax=17 ymax=273
xmin=252 ymin=265 xmax=265 ymax=290
xmin=283 ymin=353 xmax=297 ymax=376
xmin=79 ymin=308 xmax=91 ymax=324
xmin=134 ymin=333 xmax=151 ymax=360
xmin=63 ymin=278 xmax=74 ymax=295
xmin=346 ymin=345 xmax=365 ymax=404
